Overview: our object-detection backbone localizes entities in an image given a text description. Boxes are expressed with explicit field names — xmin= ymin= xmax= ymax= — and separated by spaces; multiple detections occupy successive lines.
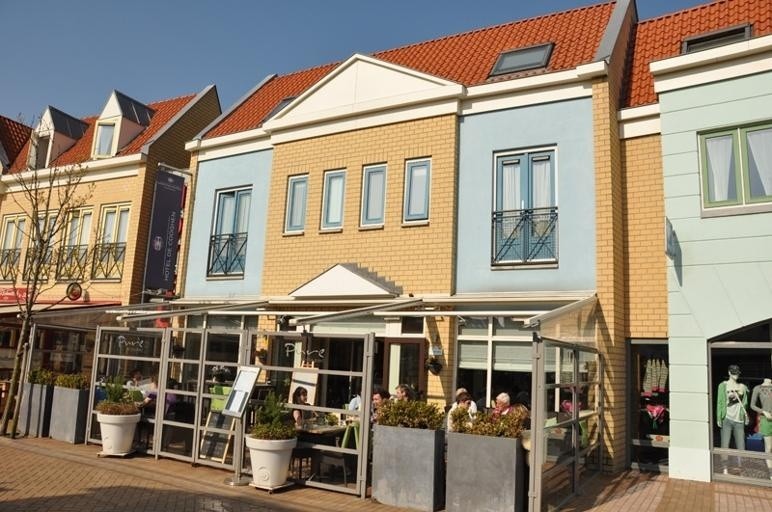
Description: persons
xmin=750 ymin=377 xmax=771 ymax=480
xmin=716 ymin=364 xmax=749 ymax=476
xmin=293 ymin=383 xmax=531 ymax=482
xmin=127 ymin=370 xmax=144 ymax=394
xmin=135 ymin=376 xmax=181 ymax=444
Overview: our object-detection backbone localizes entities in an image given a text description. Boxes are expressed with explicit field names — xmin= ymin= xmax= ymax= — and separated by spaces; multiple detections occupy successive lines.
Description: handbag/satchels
xmin=744 ymin=412 xmax=751 ymax=427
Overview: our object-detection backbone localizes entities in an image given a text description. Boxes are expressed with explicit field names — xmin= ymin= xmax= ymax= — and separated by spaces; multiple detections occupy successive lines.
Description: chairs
xmin=354 ymin=425 xmax=360 ymax=449
xmin=306 ymin=424 xmax=353 ymax=487
xmin=146 ymin=401 xmax=195 ymax=455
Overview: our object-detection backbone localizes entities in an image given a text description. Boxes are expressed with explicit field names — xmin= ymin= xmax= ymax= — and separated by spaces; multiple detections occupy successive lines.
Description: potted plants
xmin=97 ymin=383 xmax=141 ymax=455
xmin=246 ymin=392 xmax=298 ymax=489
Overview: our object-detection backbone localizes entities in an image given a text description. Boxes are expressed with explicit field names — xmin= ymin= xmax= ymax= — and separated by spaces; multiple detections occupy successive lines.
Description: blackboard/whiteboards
xmin=199 ymin=411 xmax=235 ymax=462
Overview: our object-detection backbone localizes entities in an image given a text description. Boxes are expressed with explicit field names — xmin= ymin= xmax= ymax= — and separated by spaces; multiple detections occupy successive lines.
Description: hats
xmin=728 ymin=364 xmax=742 ymax=375
